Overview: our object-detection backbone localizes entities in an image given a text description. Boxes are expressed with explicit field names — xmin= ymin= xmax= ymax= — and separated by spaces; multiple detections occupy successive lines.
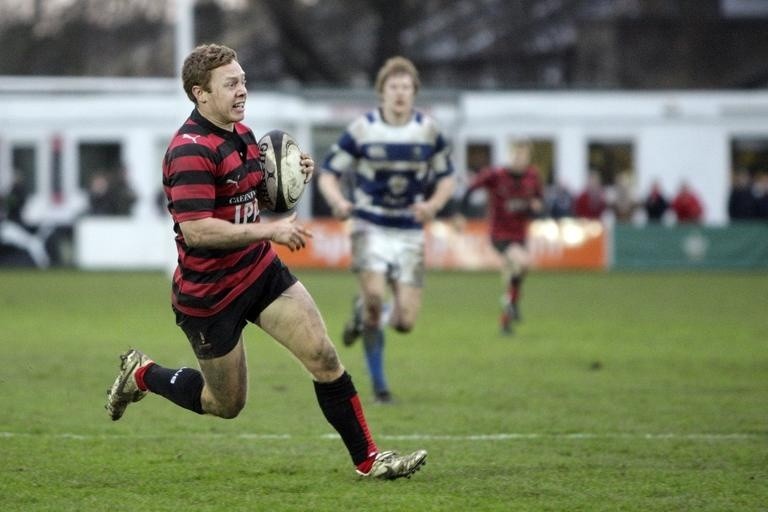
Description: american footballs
xmin=257 ymin=129 xmax=307 ymax=212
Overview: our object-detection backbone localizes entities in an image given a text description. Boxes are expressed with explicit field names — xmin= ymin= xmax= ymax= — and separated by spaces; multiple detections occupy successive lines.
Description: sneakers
xmin=374 ymin=385 xmax=391 ymax=403
xmin=354 ymin=450 xmax=428 ymax=481
xmin=104 ymin=349 xmax=156 ymax=421
xmin=344 ymin=296 xmax=365 ymax=346
xmin=500 ymin=300 xmax=520 ymax=334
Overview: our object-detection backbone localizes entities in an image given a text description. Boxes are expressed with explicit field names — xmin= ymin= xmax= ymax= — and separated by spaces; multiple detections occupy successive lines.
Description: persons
xmin=318 ymin=56 xmax=455 ymax=404
xmin=462 ymin=134 xmax=545 ymax=337
xmin=105 ymin=44 xmax=428 ymax=480
xmin=550 ymin=168 xmax=703 ymax=223
